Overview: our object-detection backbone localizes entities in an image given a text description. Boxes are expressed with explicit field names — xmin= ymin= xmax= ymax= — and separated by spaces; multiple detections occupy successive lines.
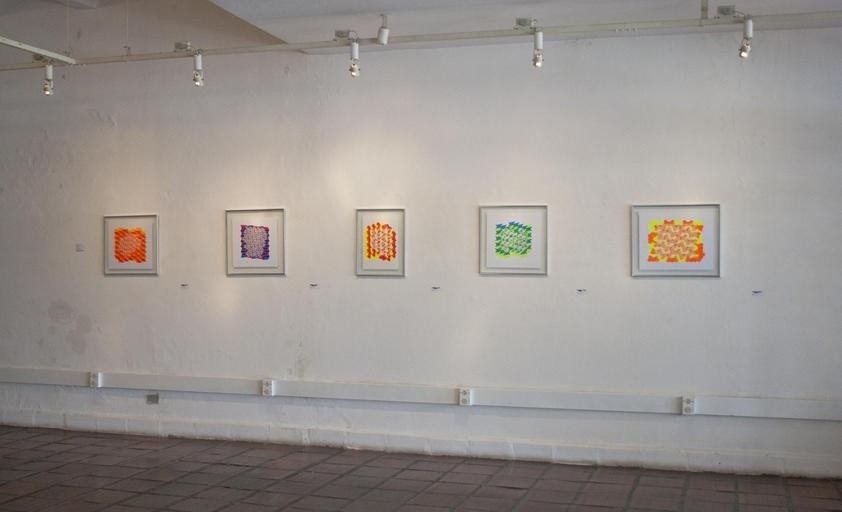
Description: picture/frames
xmin=103 ymin=214 xmax=158 ymax=276
xmin=356 ymin=209 xmax=405 ymax=277
xmin=225 ymin=208 xmax=285 ymax=275
xmin=630 ymin=204 xmax=721 ymax=278
xmin=480 ymin=206 xmax=548 ymax=275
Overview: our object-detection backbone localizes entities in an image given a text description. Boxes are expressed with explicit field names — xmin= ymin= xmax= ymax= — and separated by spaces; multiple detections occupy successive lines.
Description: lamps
xmin=349 ymin=42 xmax=360 ymax=77
xmin=739 ymin=18 xmax=754 ymax=58
xmin=377 ymin=25 xmax=389 ymax=45
xmin=43 ymin=66 xmax=54 ymax=96
xmin=532 ymin=32 xmax=545 ymax=66
xmin=193 ymin=54 xmax=205 ymax=87
